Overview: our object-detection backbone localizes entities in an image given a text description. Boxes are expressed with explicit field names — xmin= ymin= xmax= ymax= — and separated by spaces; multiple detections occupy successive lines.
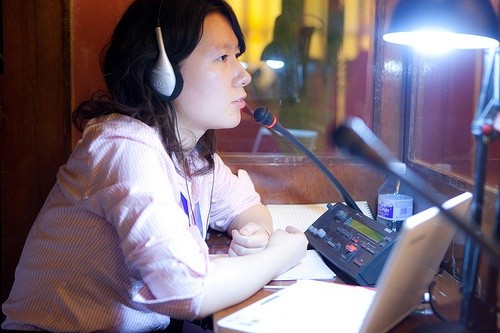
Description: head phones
xmin=142 ymin=0 xmax=184 ymax=101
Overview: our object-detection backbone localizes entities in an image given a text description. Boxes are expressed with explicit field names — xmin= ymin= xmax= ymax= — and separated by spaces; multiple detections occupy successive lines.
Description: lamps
xmin=383 ymin=0 xmax=500 ymax=56
xmin=260 ymin=13 xmax=325 ymax=71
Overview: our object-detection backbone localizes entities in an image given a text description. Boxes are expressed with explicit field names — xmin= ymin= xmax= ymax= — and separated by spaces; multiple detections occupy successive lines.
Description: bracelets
xmin=264 ymin=228 xmax=272 ymax=241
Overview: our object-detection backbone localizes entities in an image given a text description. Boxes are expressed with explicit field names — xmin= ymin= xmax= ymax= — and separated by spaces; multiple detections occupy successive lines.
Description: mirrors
xmin=217 ymin=0 xmax=384 ymax=165
xmin=398 ymin=53 xmax=499 ymax=205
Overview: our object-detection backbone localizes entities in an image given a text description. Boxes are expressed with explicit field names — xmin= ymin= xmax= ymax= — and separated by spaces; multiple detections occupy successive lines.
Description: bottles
xmin=376 ymin=162 xmax=413 ymax=232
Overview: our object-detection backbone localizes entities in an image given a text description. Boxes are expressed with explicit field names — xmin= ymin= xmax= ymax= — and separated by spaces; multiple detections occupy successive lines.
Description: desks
xmin=205 ymin=225 xmax=500 ymax=333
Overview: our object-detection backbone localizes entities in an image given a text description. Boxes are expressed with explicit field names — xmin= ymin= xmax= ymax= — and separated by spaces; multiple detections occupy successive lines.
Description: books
xmin=263 ymin=200 xmax=375 ymax=237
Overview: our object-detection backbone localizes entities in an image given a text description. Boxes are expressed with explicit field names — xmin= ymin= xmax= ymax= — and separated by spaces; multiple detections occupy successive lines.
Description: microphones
xmin=328 ymin=116 xmax=500 ymax=262
xmin=254 ymin=107 xmax=363 ymax=214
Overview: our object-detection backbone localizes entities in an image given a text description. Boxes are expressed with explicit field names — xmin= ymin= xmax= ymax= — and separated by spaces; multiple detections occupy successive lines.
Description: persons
xmin=260 ymin=12 xmax=305 ymax=107
xmin=0 ymin=0 xmax=310 ymax=333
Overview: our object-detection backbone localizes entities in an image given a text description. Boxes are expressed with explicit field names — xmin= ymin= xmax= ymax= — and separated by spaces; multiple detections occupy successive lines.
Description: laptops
xmin=216 ymin=190 xmax=472 ymax=333
